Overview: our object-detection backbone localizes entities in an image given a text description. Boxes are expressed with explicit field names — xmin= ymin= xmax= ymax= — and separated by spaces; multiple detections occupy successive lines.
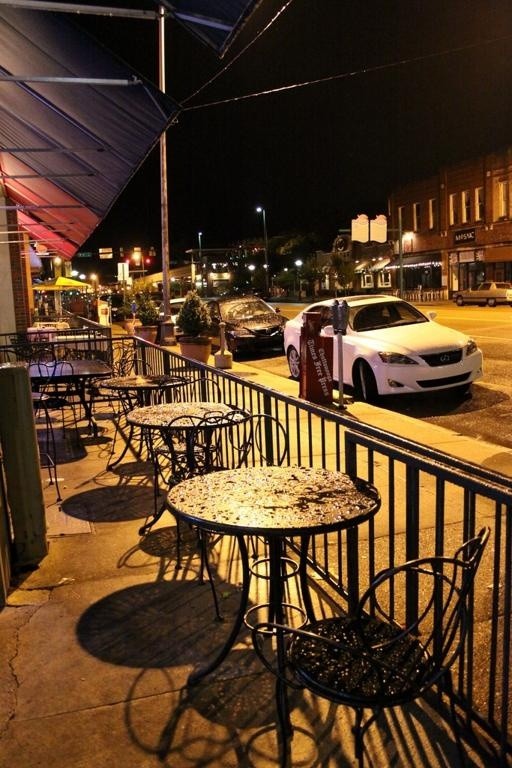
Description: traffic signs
xmin=77 ymin=252 xmax=92 ymax=257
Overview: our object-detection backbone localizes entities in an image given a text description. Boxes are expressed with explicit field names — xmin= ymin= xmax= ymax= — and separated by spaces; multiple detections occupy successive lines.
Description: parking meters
xmin=329 ymin=299 xmax=351 ymax=410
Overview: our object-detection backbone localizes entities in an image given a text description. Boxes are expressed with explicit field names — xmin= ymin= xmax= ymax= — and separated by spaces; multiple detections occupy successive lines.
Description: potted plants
xmin=133 ymin=296 xmax=165 ymax=343
xmin=173 ymin=286 xmax=215 ymax=365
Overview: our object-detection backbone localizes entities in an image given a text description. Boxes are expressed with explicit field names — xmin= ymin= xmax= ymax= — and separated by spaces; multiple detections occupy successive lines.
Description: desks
xmin=165 ymin=463 xmax=383 ymax=739
xmin=127 ymin=401 xmax=252 ymax=569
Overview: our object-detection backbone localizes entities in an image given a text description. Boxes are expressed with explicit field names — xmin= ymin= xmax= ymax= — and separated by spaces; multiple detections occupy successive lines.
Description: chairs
xmin=0 ymin=316 xmax=224 ymax=486
xmin=162 ymin=408 xmax=289 ymax=622
xmin=251 ymin=522 xmax=496 ymax=767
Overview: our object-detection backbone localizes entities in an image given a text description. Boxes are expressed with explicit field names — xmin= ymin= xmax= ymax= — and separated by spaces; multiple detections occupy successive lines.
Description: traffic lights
xmin=145 ymin=257 xmax=151 ymax=265
xmin=125 ymin=257 xmax=131 ymax=265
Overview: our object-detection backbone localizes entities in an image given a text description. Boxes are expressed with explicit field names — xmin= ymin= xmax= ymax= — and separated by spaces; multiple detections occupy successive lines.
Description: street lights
xmin=256 ymin=205 xmax=270 ymax=303
xmin=198 ymin=232 xmax=203 ymax=258
xmin=295 ymin=260 xmax=303 ymax=301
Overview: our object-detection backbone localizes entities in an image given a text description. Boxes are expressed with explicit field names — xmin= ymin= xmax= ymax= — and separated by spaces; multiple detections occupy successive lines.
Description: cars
xmin=452 ymin=280 xmax=512 ymax=307
xmin=77 ymin=292 xmax=164 ymax=318
xmin=156 ymin=297 xmax=187 ymax=341
xmin=283 ymin=295 xmax=483 ymax=408
xmin=203 ymin=298 xmax=291 ymax=361
